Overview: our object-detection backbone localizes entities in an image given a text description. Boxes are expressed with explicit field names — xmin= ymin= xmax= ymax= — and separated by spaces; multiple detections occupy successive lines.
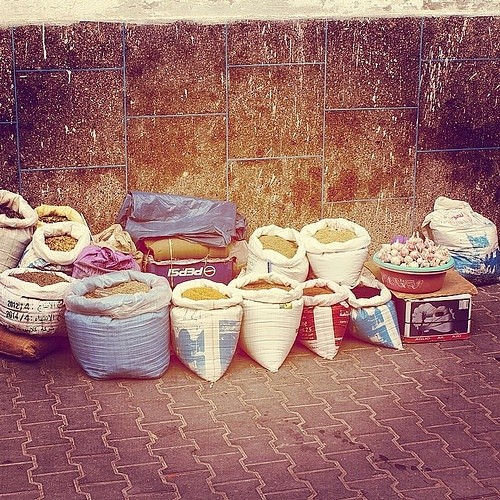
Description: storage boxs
xmin=142 ymin=253 xmax=238 ymax=291
xmin=389 ymin=293 xmax=473 ymax=344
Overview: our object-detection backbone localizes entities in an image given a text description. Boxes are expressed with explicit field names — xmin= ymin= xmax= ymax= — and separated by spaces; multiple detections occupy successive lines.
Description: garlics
xmin=377 ymin=231 xmax=451 ymax=267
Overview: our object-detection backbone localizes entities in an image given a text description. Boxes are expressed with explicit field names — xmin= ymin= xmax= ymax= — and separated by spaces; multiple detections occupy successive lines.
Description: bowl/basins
xmin=373 ymin=251 xmax=455 ymax=293
